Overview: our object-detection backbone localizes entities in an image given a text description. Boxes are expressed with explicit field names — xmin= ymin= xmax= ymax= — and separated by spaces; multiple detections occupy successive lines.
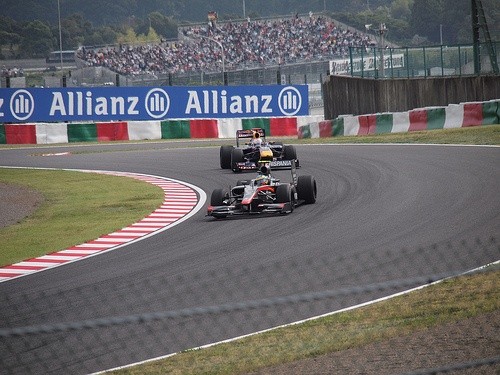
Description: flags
xmin=208 ymin=11 xmax=215 ymax=19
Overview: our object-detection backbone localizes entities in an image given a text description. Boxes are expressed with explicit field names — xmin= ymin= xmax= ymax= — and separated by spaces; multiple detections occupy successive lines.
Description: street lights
xmin=188 ymin=32 xmax=225 ymax=86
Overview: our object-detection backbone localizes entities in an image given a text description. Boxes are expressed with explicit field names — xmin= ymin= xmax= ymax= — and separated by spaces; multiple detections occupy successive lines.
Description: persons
xmin=253 ymin=166 xmax=273 ymax=185
xmin=252 ymin=133 xmax=264 ymax=147
xmin=0 ymin=10 xmax=405 ymax=83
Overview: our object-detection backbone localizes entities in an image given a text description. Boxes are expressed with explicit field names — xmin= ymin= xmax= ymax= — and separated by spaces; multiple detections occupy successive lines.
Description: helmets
xmin=256 ymin=175 xmax=269 ymax=185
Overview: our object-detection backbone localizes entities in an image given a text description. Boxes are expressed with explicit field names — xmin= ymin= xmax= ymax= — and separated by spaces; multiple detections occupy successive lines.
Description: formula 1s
xmin=205 ymin=162 xmax=318 ymax=217
xmin=219 ymin=128 xmax=301 ymax=173
